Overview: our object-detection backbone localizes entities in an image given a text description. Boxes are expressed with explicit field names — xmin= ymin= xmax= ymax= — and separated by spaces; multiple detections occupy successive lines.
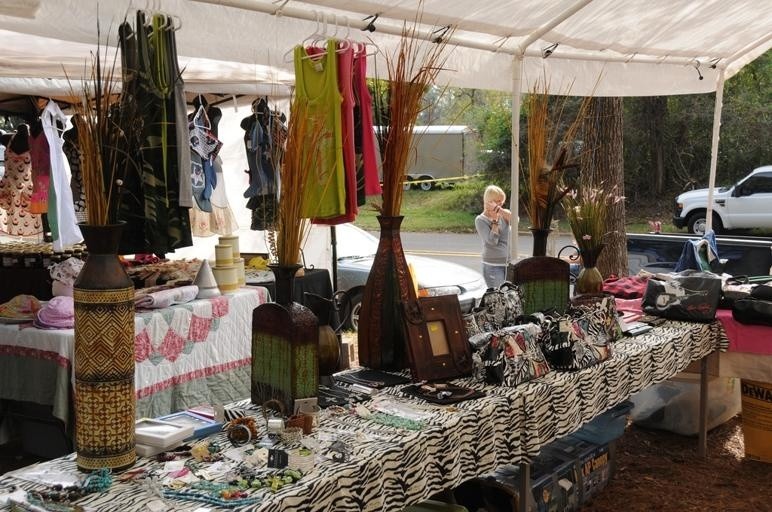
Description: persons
xmin=474 ymin=185 xmax=520 ymax=290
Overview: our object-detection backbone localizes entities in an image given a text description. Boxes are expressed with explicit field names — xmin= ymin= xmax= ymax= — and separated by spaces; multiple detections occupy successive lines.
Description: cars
xmin=296 ymin=220 xmax=490 ymax=333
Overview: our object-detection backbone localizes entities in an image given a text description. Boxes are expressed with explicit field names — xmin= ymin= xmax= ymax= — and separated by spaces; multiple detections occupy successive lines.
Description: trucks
xmin=370 ymin=125 xmax=481 ymax=192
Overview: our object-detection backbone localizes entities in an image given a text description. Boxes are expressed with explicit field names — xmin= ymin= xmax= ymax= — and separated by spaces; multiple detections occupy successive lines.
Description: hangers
xmin=193 ymin=93 xmax=212 ymax=131
xmin=281 ymin=10 xmax=380 ymax=64
xmin=250 ymin=95 xmax=282 ymax=118
xmin=118 ymin=0 xmax=183 ymax=43
xmin=43 ymin=95 xmax=66 ymax=133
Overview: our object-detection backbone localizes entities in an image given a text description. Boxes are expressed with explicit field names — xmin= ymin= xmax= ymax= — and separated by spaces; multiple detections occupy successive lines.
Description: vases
xmin=359 ymin=217 xmax=418 ymax=369
xmin=512 ymin=229 xmax=570 ymax=313
xmin=72 ymin=221 xmax=137 ymax=472
xmin=557 ymin=245 xmax=604 ymax=297
xmin=250 ymin=263 xmax=320 ymax=418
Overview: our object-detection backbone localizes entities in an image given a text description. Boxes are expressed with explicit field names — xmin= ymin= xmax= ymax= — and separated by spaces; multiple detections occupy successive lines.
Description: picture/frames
xmin=400 ymin=294 xmax=477 ymax=380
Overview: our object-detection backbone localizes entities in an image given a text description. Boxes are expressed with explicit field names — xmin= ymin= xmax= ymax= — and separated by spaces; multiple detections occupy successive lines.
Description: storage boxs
xmin=410 ymin=501 xmax=467 ymax=510
xmin=573 ymin=400 xmax=635 ymax=444
xmin=740 ymin=378 xmax=771 ymax=463
xmin=631 ymin=373 xmax=741 ymax=435
xmin=454 ymin=433 xmax=615 ymax=510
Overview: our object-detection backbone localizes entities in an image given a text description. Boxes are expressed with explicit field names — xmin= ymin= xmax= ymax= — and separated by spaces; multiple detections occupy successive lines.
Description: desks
xmin=1 ymin=312 xmax=730 ymax=507
xmin=615 ymin=276 xmax=770 ymax=381
xmin=247 ymin=269 xmax=342 ymax=340
xmin=1 ymin=286 xmax=272 ymax=457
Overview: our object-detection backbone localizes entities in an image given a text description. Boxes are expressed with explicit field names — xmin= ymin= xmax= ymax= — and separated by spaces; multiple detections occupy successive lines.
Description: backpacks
xmin=639 ymin=276 xmax=720 ymax=324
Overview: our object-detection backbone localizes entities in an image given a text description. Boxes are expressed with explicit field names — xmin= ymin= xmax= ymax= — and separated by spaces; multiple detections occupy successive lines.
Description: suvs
xmin=671 ymin=165 xmax=772 ymax=237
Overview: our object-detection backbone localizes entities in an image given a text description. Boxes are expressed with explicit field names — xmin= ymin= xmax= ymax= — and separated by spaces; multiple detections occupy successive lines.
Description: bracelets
xmin=491 ymin=220 xmax=499 ymax=225
xmin=493 ymin=204 xmax=501 ymax=213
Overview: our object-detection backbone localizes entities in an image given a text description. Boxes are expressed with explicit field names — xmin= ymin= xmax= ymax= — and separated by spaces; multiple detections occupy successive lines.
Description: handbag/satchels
xmin=461 ymin=305 xmax=496 ymax=358
xmin=540 ymin=315 xmax=610 ymax=371
xmin=731 ymin=284 xmax=772 ymax=327
xmin=484 ymin=286 xmax=525 ymax=329
xmin=481 ymin=328 xmax=551 ymax=389
xmin=565 ymin=292 xmax=623 ymax=343
xmin=719 ymin=282 xmax=759 ymax=310
